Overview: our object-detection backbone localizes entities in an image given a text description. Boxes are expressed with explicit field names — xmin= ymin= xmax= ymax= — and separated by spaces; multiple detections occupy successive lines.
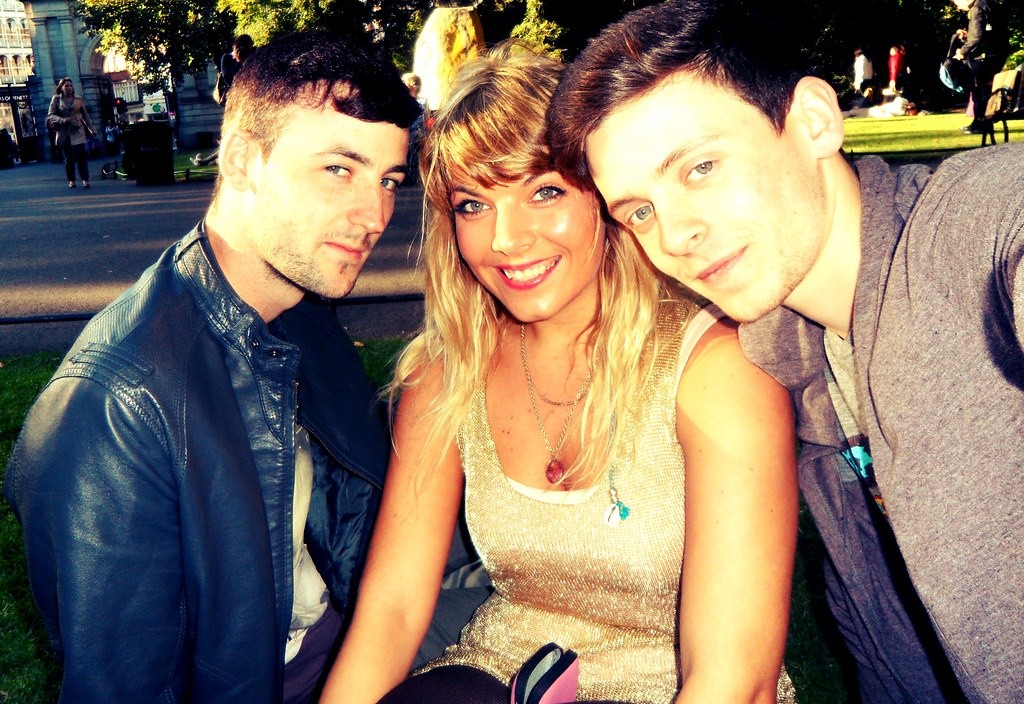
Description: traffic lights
xmin=114 ymin=97 xmax=129 ymax=114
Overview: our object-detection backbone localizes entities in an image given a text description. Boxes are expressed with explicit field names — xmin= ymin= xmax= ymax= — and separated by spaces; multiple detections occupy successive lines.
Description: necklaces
xmin=520 ymin=321 xmax=605 ymax=483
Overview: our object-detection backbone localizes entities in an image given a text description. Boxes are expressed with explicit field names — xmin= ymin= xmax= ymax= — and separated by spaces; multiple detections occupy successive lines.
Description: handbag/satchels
xmin=45 ymin=94 xmax=61 ymax=130
xmin=212 ymin=72 xmax=230 ymax=106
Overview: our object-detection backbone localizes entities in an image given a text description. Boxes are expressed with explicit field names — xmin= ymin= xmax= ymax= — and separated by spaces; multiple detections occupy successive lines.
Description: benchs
xmin=978 ymin=66 xmax=1024 ymax=148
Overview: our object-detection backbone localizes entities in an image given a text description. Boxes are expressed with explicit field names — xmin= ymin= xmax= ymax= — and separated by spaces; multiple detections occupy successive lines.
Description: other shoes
xmin=962 ymin=122 xmax=979 ymax=134
xmin=190 ymin=157 xmax=200 ymax=167
xmin=69 ymin=181 xmax=76 ymax=188
xmin=196 ymin=154 xmax=201 ymax=160
xmin=82 ymin=179 xmax=90 ymax=189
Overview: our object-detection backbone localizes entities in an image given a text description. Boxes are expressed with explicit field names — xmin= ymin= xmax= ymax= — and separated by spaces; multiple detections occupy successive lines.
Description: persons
xmin=839 ymin=0 xmax=997 ymax=134
xmin=105 ymin=119 xmax=120 ymax=142
xmin=2 ymin=28 xmax=489 ymax=704
xmin=45 ymin=77 xmax=96 ymax=189
xmin=545 ymin=0 xmax=1024 ymax=704
xmin=317 ymin=36 xmax=801 ymax=704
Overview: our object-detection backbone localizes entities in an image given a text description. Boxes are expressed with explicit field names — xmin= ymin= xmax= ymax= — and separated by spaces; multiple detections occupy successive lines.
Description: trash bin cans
xmin=130 ymin=118 xmax=176 ymax=186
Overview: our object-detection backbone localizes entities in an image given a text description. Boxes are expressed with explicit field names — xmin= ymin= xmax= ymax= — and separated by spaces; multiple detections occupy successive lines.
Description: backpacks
xmin=939 ymin=33 xmax=975 ymax=94
